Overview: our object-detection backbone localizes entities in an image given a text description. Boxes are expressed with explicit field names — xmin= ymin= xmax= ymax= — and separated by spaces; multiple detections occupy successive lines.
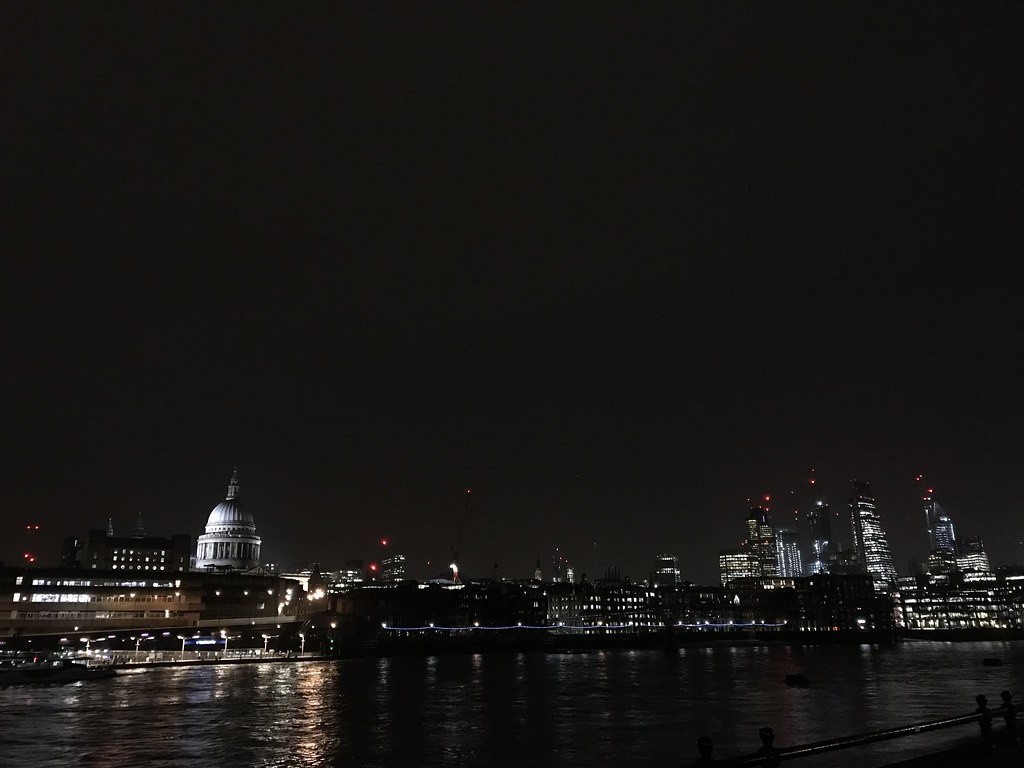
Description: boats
xmin=81 ymin=664 xmax=118 ymax=678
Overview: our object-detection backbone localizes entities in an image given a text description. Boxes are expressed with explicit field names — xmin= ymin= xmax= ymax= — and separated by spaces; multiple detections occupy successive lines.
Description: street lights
xmin=130 ymin=636 xmax=154 ymax=661
xmin=177 ymin=636 xmax=200 ymax=660
xmin=221 ymin=635 xmax=231 ymax=652
xmin=299 ymin=634 xmax=305 ymax=656
xmin=262 ymin=635 xmax=271 ymax=653
xmin=80 ymin=638 xmax=106 ymax=663
